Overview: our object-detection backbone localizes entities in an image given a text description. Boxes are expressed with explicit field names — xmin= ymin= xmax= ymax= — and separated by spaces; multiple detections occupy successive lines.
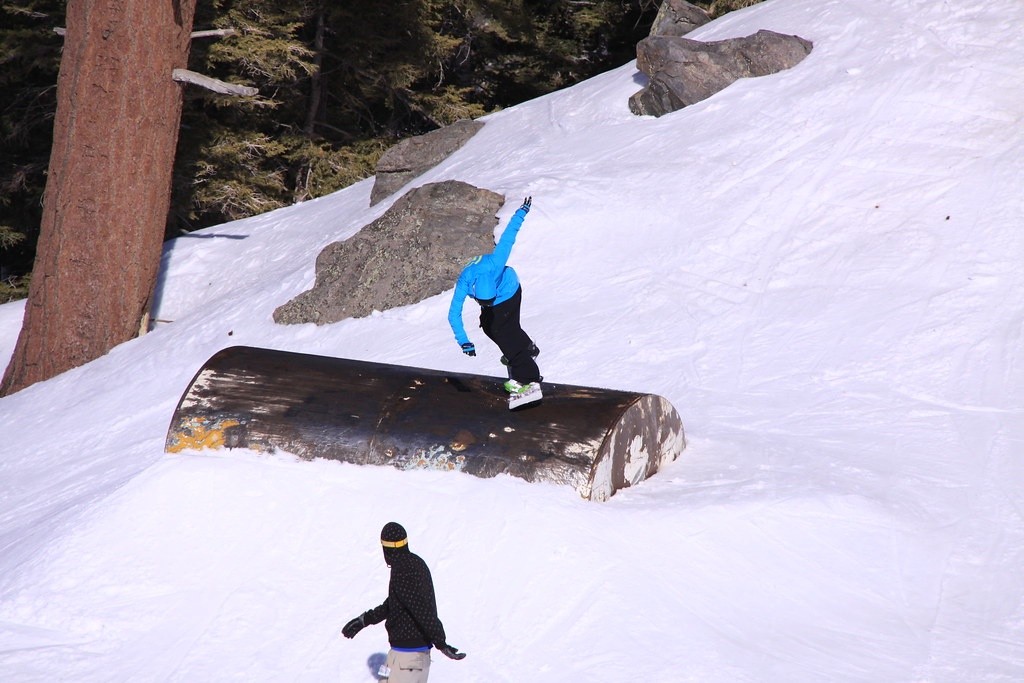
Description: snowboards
xmin=506 ymin=356 xmax=544 ymax=410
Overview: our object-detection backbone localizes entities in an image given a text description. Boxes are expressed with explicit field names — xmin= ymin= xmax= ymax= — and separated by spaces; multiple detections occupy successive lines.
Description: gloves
xmin=341 ymin=617 xmax=364 ymax=639
xmin=441 ymin=645 xmax=466 ymax=660
xmin=461 ymin=343 xmax=477 ymax=357
xmin=520 ymin=196 xmax=534 ymax=213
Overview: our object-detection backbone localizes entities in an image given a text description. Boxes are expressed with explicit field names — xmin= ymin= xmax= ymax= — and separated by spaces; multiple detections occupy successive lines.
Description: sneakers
xmin=500 ymin=341 xmax=540 ymax=366
xmin=502 ymin=379 xmax=526 ymax=393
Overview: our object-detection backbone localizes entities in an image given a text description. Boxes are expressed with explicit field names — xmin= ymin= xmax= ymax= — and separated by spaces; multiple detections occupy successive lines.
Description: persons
xmin=341 ymin=522 xmax=466 ymax=683
xmin=447 ymin=196 xmax=544 ymax=409
